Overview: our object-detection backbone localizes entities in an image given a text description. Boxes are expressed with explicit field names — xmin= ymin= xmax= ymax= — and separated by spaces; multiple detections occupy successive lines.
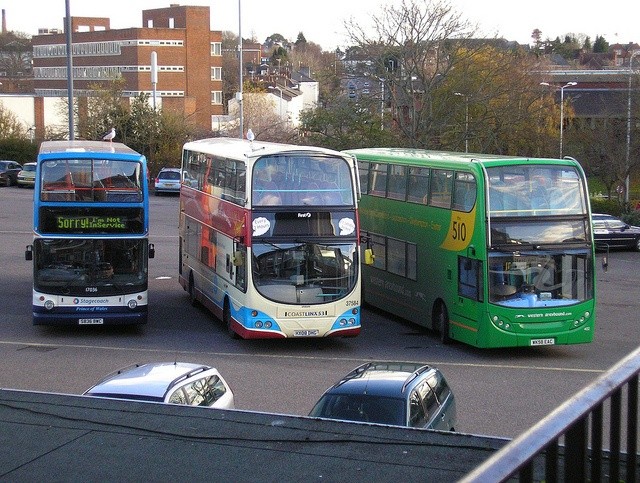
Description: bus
xmin=25 ymin=142 xmax=156 ymax=328
xmin=340 ymin=147 xmax=597 ymax=348
xmin=179 ymin=137 xmax=375 ymax=340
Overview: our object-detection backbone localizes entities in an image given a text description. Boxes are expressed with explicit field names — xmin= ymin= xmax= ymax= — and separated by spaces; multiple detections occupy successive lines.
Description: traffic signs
xmin=616 ymin=185 xmax=624 ymax=194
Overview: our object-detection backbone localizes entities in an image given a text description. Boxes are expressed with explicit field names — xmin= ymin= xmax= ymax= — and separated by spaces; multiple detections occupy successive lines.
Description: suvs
xmin=307 ymin=359 xmax=457 ymax=433
xmin=81 ymin=346 xmax=235 ymax=411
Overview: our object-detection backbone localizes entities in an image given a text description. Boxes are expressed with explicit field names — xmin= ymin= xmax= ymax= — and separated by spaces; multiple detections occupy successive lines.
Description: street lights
xmin=269 ymin=85 xmax=299 ymax=123
xmin=24 ymin=127 xmax=37 ymax=135
xmin=236 ymin=0 xmax=245 ymax=138
xmin=363 ymin=71 xmax=417 ymax=130
xmin=151 ymin=51 xmax=157 ymax=109
xmin=455 ymin=91 xmax=482 ymax=153
xmin=540 ymin=80 xmax=577 ymax=158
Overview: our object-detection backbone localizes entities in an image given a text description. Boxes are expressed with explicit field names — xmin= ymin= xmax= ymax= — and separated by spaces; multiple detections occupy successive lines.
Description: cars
xmin=155 ymin=167 xmax=181 ymax=195
xmin=591 ymin=213 xmax=640 ymax=255
xmin=0 ymin=161 xmax=22 ymax=188
xmin=17 ymin=163 xmax=38 ymax=187
